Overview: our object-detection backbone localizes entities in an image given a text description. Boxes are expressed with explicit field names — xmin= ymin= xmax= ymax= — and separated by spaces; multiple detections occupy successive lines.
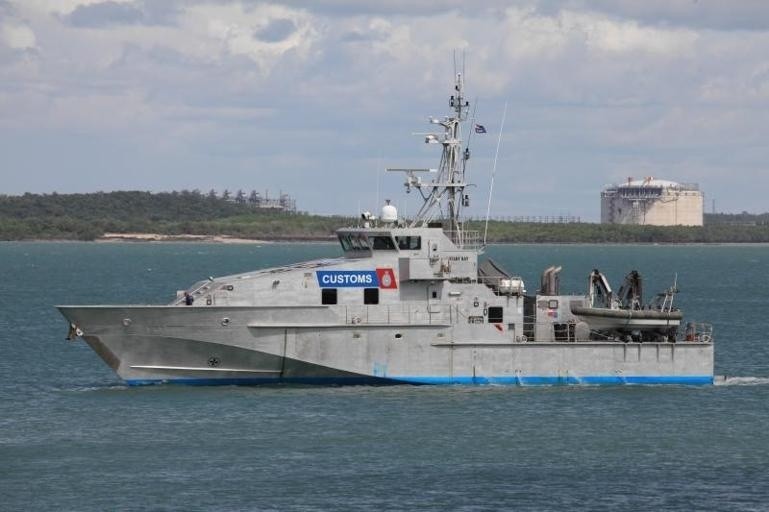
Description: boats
xmin=53 ymin=45 xmax=715 ymax=388
xmin=570 ymin=304 xmax=682 ymax=330
